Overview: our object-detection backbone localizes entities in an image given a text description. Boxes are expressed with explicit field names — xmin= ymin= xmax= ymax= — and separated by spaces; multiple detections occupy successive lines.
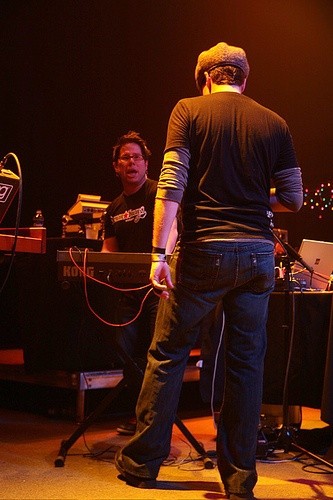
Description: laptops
xmin=290 ymin=239 xmax=333 ymax=290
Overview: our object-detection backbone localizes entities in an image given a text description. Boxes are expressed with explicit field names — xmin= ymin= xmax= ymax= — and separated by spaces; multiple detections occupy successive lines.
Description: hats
xmin=194 ymin=42 xmax=249 ymax=94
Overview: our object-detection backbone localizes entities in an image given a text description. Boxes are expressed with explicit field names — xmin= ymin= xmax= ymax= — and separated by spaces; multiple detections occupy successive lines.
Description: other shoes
xmin=115 ymin=447 xmax=155 ymax=489
xmin=117 ymin=422 xmax=136 ymax=434
xmin=226 ymin=492 xmax=253 ymax=500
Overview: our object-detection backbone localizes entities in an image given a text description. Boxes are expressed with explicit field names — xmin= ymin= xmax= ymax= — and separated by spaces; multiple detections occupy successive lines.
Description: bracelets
xmin=152 ymin=246 xmax=165 ymax=254
xmin=151 ymin=254 xmax=166 ymax=262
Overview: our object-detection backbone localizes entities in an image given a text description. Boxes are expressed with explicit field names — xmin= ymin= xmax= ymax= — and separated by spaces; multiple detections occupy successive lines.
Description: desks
xmin=266 ymin=293 xmax=333 ymax=428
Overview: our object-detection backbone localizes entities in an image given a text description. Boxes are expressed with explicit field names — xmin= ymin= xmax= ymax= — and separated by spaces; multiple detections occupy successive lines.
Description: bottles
xmin=274 ymin=232 xmax=291 ymax=291
xmin=33 ymin=210 xmax=43 ymax=226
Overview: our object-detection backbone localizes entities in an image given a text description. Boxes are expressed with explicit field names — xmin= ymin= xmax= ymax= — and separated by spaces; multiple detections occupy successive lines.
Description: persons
xmin=101 ymin=130 xmax=178 ymax=435
xmin=114 ymin=41 xmax=302 ymax=500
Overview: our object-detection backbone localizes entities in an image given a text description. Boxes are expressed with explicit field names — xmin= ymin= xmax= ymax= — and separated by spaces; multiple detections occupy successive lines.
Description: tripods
xmin=257 ymin=234 xmax=333 ymax=469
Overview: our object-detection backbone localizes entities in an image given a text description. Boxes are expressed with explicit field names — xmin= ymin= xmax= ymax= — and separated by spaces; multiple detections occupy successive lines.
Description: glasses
xmin=116 ymin=154 xmax=144 ymax=162
